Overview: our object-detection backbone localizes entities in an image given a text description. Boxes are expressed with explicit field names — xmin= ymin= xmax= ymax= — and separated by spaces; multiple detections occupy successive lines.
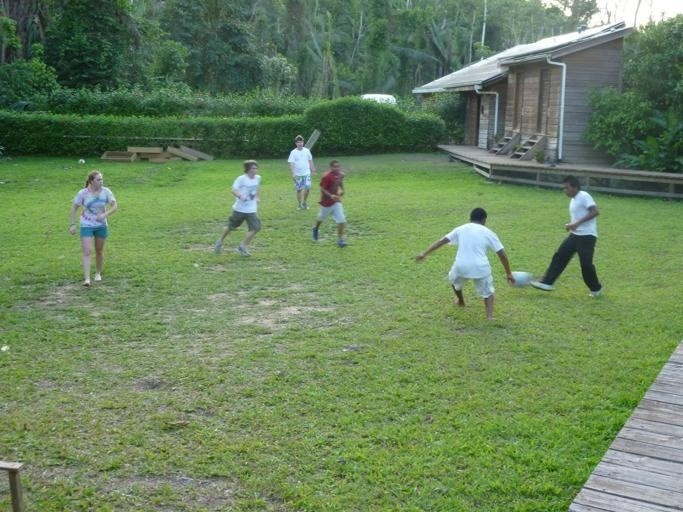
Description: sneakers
xmin=529 ymin=281 xmax=554 ymax=292
xmin=236 ymin=245 xmax=251 ymax=256
xmin=312 ymin=226 xmax=319 ymax=240
xmin=589 ymin=286 xmax=604 ymax=298
xmin=83 ymin=279 xmax=89 ymax=285
xmin=337 ymin=239 xmax=347 ymax=247
xmin=95 ymin=275 xmax=101 ymax=281
xmin=215 ymin=240 xmax=223 ymax=253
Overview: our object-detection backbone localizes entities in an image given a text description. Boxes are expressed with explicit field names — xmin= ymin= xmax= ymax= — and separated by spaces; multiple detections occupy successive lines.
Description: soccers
xmin=511 ymin=271 xmax=532 ymax=287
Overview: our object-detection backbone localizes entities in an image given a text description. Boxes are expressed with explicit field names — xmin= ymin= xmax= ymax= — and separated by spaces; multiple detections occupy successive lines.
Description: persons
xmin=410 ymin=206 xmax=514 ymax=324
xmin=310 ymin=159 xmax=349 ymax=248
xmin=211 ymin=158 xmax=264 ymax=259
xmin=286 ymin=134 xmax=318 ymax=212
xmin=529 ymin=173 xmax=605 ymax=297
xmin=66 ymin=169 xmax=118 ymax=288
